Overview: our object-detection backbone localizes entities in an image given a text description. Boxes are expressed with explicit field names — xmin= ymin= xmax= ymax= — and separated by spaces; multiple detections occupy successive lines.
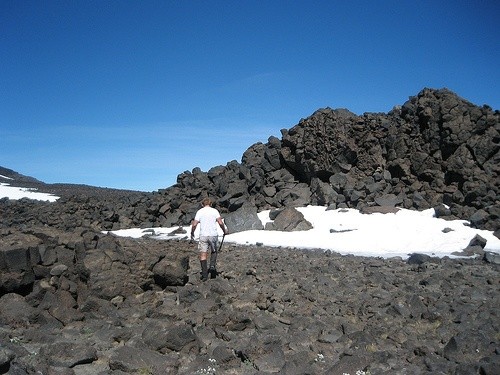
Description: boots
xmin=201 ymin=253 xmax=216 ymax=279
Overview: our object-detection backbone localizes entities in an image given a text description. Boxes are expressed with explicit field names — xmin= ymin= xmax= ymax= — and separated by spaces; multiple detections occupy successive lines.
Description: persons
xmin=192 ymin=198 xmax=228 ymax=278
